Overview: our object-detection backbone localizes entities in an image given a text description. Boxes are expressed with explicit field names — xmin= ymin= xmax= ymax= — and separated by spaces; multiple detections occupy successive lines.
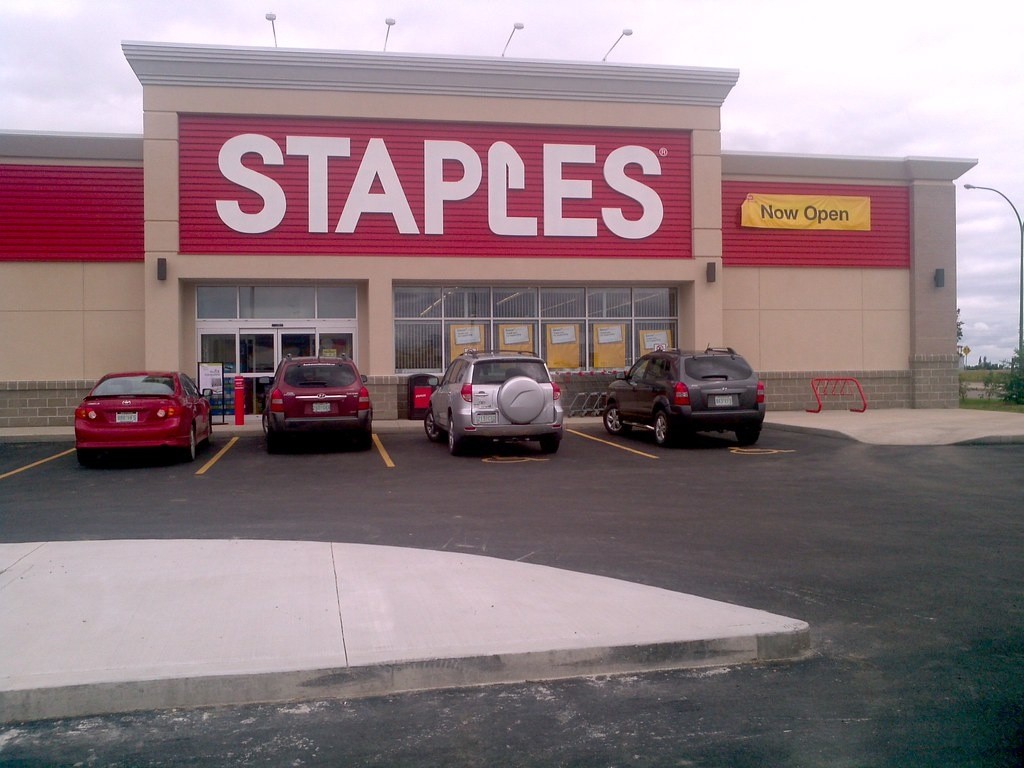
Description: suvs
xmin=602 ymin=347 xmax=767 ymax=447
xmin=261 ymin=352 xmax=374 ymax=450
xmin=424 ymin=348 xmax=565 ymax=454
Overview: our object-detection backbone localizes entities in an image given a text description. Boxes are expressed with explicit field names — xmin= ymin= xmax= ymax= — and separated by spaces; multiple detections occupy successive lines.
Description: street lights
xmin=963 ymin=184 xmax=1024 ymax=395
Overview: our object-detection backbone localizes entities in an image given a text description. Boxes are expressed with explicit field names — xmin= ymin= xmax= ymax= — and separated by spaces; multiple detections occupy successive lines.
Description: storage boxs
xmin=208 ymin=362 xmax=235 ymax=415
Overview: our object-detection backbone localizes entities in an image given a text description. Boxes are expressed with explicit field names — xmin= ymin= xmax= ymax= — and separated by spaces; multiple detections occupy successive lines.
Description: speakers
xmin=935 ymin=268 xmax=944 ymax=286
xmin=158 ymin=258 xmax=166 ymax=280
xmin=707 ymin=262 xmax=715 ymax=282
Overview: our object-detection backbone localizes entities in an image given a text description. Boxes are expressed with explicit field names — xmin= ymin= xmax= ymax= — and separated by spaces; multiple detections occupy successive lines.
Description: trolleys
xmin=549 ymin=368 xmax=630 ymax=417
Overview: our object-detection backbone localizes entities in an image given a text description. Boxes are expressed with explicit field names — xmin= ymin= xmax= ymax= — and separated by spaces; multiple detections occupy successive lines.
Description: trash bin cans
xmin=407 ymin=374 xmax=440 ymax=420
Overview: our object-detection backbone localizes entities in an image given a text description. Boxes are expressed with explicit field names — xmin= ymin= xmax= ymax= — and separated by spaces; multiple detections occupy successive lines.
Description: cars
xmin=73 ymin=370 xmax=214 ymax=463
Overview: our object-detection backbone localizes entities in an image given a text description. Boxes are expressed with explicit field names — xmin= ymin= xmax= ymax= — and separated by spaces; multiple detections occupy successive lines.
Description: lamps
xmin=265 ymin=12 xmax=277 ymax=47
xmin=383 ymin=18 xmax=395 ymax=52
xmin=501 ymin=23 xmax=525 ymax=59
xmin=603 ymin=29 xmax=633 ymax=61
